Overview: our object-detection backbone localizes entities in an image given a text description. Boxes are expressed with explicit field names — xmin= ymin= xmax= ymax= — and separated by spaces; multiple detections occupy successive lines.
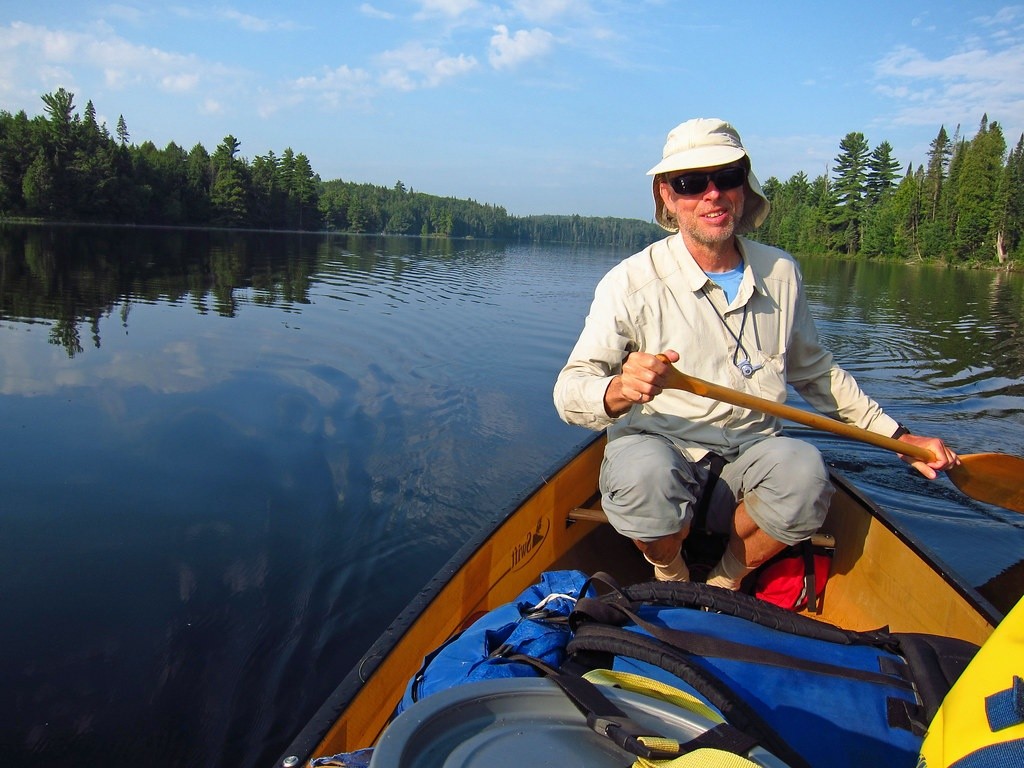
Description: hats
xmin=646 ymin=117 xmax=770 ymax=233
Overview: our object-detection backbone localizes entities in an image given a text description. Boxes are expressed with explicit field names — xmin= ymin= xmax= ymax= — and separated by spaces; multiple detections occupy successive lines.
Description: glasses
xmin=666 ymin=166 xmax=749 ymax=196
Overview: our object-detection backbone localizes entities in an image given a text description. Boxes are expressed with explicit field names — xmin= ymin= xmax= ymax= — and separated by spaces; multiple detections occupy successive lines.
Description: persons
xmin=554 ymin=119 xmax=962 ymax=616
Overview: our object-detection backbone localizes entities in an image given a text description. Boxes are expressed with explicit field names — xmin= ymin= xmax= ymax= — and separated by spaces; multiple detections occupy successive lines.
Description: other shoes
xmin=701 ymin=605 xmax=723 ymax=614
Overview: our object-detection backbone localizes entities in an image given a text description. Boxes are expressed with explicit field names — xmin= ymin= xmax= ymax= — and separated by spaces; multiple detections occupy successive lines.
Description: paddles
xmin=656 ymin=356 xmax=1024 ymax=515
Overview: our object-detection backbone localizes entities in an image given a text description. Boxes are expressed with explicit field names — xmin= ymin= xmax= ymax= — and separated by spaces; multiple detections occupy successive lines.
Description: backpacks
xmin=568 ymin=572 xmax=927 ymax=768
